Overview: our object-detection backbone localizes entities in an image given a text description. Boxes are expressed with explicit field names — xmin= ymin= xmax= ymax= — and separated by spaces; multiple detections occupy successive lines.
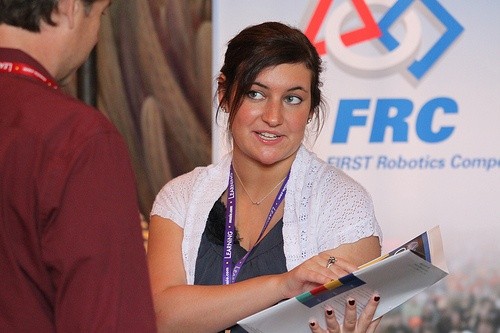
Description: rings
xmin=324 ymin=256 xmax=336 ymax=269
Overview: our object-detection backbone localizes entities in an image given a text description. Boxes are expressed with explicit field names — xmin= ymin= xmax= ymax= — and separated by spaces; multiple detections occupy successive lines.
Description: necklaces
xmin=231 ymin=161 xmax=288 ymax=204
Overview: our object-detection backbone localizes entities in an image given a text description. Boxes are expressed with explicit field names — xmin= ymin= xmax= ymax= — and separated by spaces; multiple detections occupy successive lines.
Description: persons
xmin=0 ymin=0 xmax=157 ymax=333
xmin=146 ymin=21 xmax=383 ymax=333
